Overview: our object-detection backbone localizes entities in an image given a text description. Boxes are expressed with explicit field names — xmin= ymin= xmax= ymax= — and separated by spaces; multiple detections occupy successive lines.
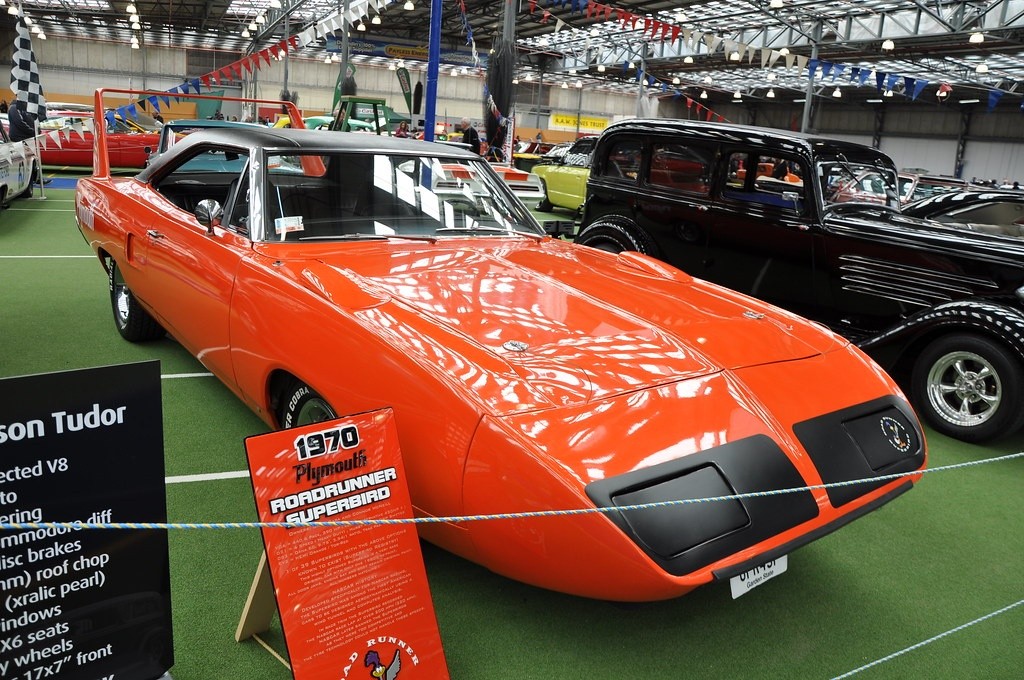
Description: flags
xmin=10 ymin=10 xmax=48 ymax=123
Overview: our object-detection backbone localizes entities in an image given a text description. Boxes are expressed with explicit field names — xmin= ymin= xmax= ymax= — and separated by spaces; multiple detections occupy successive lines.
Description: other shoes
xmin=36 ymin=178 xmax=51 ymax=184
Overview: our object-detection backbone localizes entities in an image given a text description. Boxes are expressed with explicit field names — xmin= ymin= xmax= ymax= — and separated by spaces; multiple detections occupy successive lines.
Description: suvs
xmin=530 ymin=134 xmax=602 ymax=214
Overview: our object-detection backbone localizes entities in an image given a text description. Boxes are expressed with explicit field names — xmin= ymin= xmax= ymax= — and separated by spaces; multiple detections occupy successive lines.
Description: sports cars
xmin=71 ymin=87 xmax=931 ymax=607
xmin=429 ymin=160 xmax=548 ymax=214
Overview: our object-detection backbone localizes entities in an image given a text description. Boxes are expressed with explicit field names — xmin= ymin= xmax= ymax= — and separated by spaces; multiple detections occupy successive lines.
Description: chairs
xmin=223 ymin=177 xmax=279 ymax=233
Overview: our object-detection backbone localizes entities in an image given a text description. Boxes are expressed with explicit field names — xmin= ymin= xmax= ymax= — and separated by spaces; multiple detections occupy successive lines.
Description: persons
xmin=536 ymin=133 xmax=541 ymax=142
xmin=7 ymin=95 xmax=53 ymax=184
xmin=395 ymin=121 xmax=408 ymax=138
xmin=151 ymin=109 xmax=164 ymax=124
xmin=765 ymin=156 xmax=796 ymax=174
xmin=517 ymin=136 xmax=521 ymax=141
xmin=0 ymin=101 xmax=8 ymax=113
xmin=460 ymin=117 xmax=480 ymax=155
xmin=211 ymin=109 xmax=270 ymax=125
xmin=329 ymin=108 xmax=351 ymax=132
xmin=730 ymin=153 xmax=739 ymax=178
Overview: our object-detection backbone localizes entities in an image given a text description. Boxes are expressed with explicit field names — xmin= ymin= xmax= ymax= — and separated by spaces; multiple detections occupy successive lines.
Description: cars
xmin=1 ymin=121 xmax=40 ymax=210
xmin=573 ymin=117 xmax=1024 ymax=445
xmin=37 ymin=102 xmax=188 ymax=169
xmin=830 ymin=167 xmax=970 ymax=208
xmin=434 ymin=128 xmax=550 ymax=159
xmin=145 ymin=117 xmax=304 ymax=174
